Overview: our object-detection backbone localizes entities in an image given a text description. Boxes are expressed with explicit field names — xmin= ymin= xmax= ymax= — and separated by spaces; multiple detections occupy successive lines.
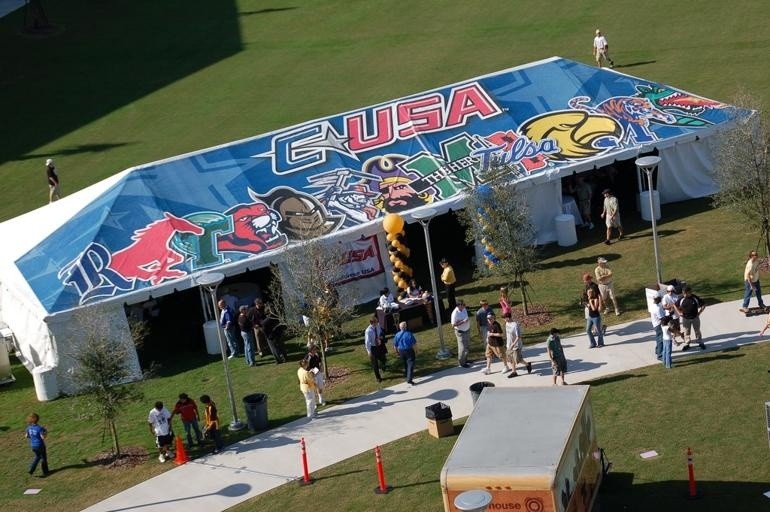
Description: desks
xmin=563 ymin=193 xmax=583 ymax=225
xmin=376 ymin=294 xmax=448 ymax=336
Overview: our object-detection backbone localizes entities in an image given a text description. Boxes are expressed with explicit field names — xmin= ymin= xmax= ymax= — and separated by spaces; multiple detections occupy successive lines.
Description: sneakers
xmin=159 ymin=456 xmax=165 ymax=463
xmin=699 ymin=344 xmax=705 ymax=349
xmin=480 ymin=368 xmax=491 ymax=374
xmin=501 ymin=365 xmax=509 ymax=374
xmin=526 ymin=362 xmax=531 ymax=373
xmin=507 ymin=372 xmax=517 ymax=378
xmin=682 ymin=345 xmax=690 ymax=352
xmin=166 ymin=452 xmax=175 ymax=459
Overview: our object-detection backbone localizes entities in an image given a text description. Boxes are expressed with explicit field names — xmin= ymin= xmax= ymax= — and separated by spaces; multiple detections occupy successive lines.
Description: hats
xmin=667 ymin=285 xmax=676 ymax=292
xmin=501 ymin=313 xmax=511 ymax=318
xmin=486 ymin=314 xmax=494 ymax=319
xmin=583 ymin=274 xmax=593 ymax=281
xmin=597 ymin=257 xmax=607 ymax=263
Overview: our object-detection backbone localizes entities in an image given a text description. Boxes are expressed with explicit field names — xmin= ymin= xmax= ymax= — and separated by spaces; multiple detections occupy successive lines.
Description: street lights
xmin=197 ymin=271 xmax=250 ymax=437
xmin=634 ymin=156 xmax=662 ymax=297
xmin=453 ymin=489 xmax=493 ymax=512
xmin=410 ymin=206 xmax=454 ymax=364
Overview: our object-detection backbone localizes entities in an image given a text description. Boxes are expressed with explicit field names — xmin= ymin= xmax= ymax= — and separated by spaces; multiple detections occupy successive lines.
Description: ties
xmin=374 ymin=327 xmax=378 ymax=339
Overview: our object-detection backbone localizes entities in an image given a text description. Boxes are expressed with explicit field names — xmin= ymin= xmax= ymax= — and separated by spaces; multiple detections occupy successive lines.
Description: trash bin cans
xmin=243 ymin=393 xmax=268 ymax=433
xmin=660 ymin=278 xmax=688 ymax=297
xmin=469 ymin=381 xmax=495 ymax=407
xmin=425 ymin=402 xmax=454 ymax=439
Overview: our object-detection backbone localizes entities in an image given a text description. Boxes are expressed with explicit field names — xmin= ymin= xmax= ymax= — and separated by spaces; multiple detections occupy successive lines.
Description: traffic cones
xmin=171 ymin=436 xmax=190 ymax=466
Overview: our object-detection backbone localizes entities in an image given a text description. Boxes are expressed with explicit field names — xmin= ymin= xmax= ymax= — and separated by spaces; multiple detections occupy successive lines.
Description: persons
xmin=22 ymin=411 xmax=50 ymax=479
xmin=217 ymin=298 xmax=289 ymax=368
xmin=360 ymin=152 xmax=437 ymax=215
xmin=362 ymin=256 xmax=533 ymax=387
xmin=569 ymin=177 xmax=595 ymax=231
xmin=146 ymin=391 xmax=224 ymax=463
xmin=297 ymin=278 xmax=341 ymax=421
xmin=759 ymin=307 xmax=770 ymax=373
xmin=581 ymin=257 xmax=624 ymax=349
xmin=648 ymin=282 xmax=706 ymax=371
xmin=545 ymin=329 xmax=567 ymax=385
xmin=44 ymin=159 xmax=63 ymax=202
xmin=593 ymin=28 xmax=616 ymax=68
xmin=599 ymin=188 xmax=625 ymax=246
xmin=742 ymin=252 xmax=765 ymax=313
